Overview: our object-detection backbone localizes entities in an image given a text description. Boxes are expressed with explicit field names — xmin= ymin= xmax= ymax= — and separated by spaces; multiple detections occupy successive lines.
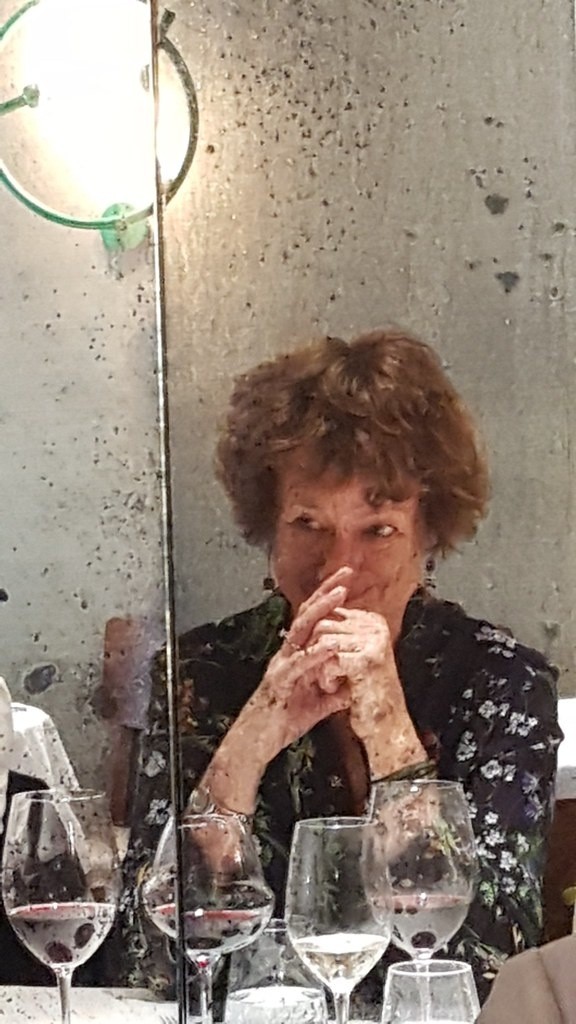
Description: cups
xmin=224 ymin=917 xmax=331 ymax=1024
xmin=381 ymin=960 xmax=483 ymax=1024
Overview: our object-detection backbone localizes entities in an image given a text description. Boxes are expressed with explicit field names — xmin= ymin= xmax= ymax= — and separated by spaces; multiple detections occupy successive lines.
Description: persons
xmin=122 ymin=331 xmax=562 ymax=1002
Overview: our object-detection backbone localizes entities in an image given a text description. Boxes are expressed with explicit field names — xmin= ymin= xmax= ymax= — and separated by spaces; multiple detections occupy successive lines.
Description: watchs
xmin=186 ymin=784 xmax=254 ymax=827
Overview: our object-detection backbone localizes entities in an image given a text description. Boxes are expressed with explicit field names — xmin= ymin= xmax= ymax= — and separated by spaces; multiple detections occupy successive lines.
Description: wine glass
xmin=283 ymin=817 xmax=392 ymax=1024
xmin=361 ymin=779 xmax=479 ymax=1024
xmin=2 ymin=790 xmax=125 ymax=1024
xmin=141 ymin=814 xmax=276 ymax=1024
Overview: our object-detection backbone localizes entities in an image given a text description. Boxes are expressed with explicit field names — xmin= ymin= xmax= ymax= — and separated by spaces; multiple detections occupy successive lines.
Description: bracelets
xmin=368 ymin=758 xmax=441 ymax=781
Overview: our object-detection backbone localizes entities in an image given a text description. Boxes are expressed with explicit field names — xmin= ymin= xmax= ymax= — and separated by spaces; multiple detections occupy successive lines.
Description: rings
xmin=276 ymin=628 xmax=303 ymax=652
